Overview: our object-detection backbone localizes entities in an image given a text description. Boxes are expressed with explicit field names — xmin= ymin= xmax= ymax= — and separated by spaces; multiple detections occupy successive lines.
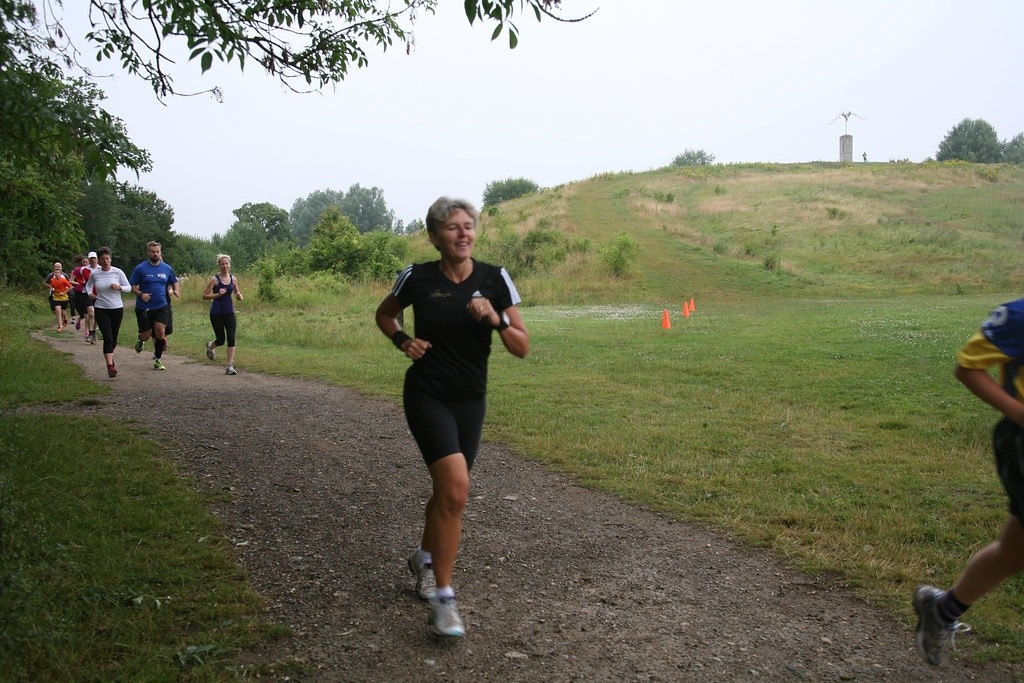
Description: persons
xmin=863 ymin=152 xmax=867 ymax=162
xmin=168 ymin=273 xmax=190 ymax=295
xmin=203 ymin=254 xmax=243 ymax=374
xmin=906 ymin=298 xmax=1024 ymax=666
xmin=129 ymin=242 xmax=179 ymax=368
xmin=43 ymin=252 xmax=101 ymax=344
xmin=375 ymin=196 xmax=529 ymax=636
xmin=85 ymin=247 xmax=131 ymax=379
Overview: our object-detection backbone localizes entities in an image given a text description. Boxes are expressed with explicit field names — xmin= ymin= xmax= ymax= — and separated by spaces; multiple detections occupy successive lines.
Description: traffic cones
xmin=682 ymin=301 xmax=690 ymax=318
xmin=662 ymin=309 xmax=671 ymax=329
xmin=689 ymin=298 xmax=695 ymax=311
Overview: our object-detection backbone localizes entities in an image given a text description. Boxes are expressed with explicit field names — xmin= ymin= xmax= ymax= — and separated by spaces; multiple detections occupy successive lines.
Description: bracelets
xmin=391 ymin=330 xmax=411 ymax=351
xmin=139 ymin=292 xmax=144 ymax=297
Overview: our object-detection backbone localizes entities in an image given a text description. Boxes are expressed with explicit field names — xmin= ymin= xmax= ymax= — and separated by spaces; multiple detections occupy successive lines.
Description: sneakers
xmin=63 ymin=320 xmax=67 ymax=327
xmin=135 ymin=334 xmax=144 ymax=353
xmin=86 ymin=335 xmax=97 ymax=344
xmin=153 ymin=360 xmax=165 ymax=369
xmin=71 ymin=320 xmax=75 ymax=324
xmin=407 ymin=547 xmax=437 ymax=600
xmin=108 ymin=363 xmax=117 ymax=378
xmin=207 ymin=342 xmax=215 ymax=360
xmin=84 ymin=331 xmax=89 ymax=337
xmin=912 ymin=583 xmax=970 ymax=667
xmin=57 ymin=327 xmax=63 ymax=332
xmin=226 ymin=366 xmax=237 ymax=375
xmin=428 ymin=597 xmax=465 ymax=636
xmin=76 ymin=322 xmax=81 ymax=330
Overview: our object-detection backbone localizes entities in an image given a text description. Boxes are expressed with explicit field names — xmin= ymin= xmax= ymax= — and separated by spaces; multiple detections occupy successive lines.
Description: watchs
xmin=495 ymin=311 xmax=509 ymax=331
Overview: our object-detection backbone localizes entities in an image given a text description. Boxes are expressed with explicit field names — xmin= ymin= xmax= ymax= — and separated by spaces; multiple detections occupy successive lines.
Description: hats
xmin=87 ymin=252 xmax=97 ymax=258
xmin=55 ymin=263 xmax=62 ymax=270
